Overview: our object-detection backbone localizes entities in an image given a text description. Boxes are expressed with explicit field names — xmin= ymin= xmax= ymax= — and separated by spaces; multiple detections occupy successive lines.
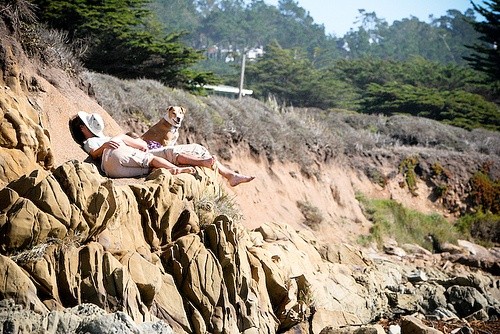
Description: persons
xmin=102 ymin=132 xmax=195 ymax=175
xmin=72 ymin=115 xmax=255 ymax=187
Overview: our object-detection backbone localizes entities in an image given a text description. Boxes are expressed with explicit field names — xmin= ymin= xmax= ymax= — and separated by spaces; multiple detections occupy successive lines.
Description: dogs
xmin=141 ymin=106 xmax=187 ymax=147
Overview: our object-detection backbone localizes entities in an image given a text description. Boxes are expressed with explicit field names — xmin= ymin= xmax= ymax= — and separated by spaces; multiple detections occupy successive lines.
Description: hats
xmin=78 ymin=111 xmax=104 ymax=137
